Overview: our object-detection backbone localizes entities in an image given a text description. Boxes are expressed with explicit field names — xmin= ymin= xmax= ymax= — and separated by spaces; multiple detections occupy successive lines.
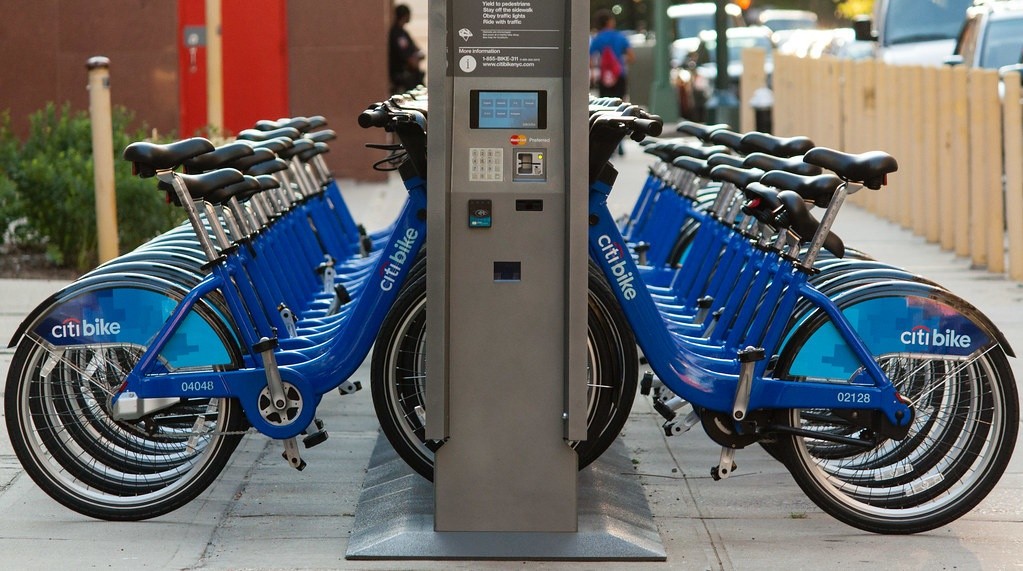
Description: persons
xmin=590 ymin=10 xmax=634 ymax=97
xmin=390 ymin=5 xmax=425 ymax=91
xmin=686 ymin=41 xmax=711 ymax=123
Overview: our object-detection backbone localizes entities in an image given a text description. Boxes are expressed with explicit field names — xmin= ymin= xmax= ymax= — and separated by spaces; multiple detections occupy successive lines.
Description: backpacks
xmin=600 ymin=46 xmax=622 ymax=88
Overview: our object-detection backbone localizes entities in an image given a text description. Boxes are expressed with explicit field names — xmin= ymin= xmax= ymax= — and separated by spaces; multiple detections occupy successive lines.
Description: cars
xmin=944 ymin=1 xmax=1023 ymax=107
xmin=617 ymin=0 xmax=971 ymax=86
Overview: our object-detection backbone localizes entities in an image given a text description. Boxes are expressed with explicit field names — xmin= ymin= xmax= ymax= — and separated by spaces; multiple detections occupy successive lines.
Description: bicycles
xmin=584 ymin=87 xmax=999 ymax=512
xmin=4 ymin=100 xmax=435 ymax=523
xmin=27 ymin=86 xmax=432 ymax=489
xmin=582 ymin=91 xmax=1022 ymax=536
